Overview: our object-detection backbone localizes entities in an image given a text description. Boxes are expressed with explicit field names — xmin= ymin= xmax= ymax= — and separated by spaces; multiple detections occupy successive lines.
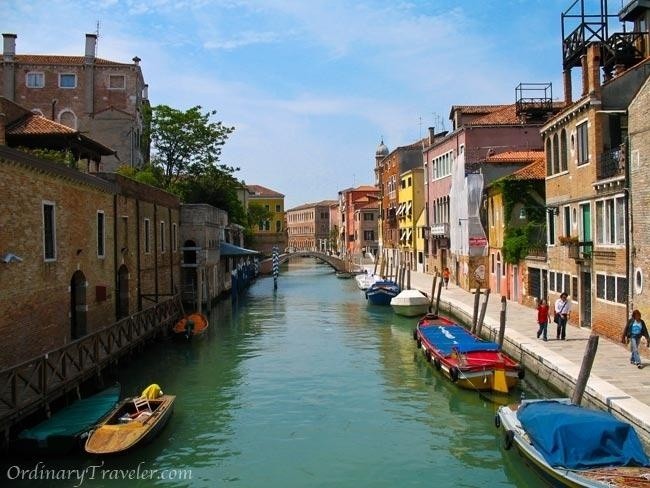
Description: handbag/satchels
xmin=553 ymin=312 xmax=560 ymax=323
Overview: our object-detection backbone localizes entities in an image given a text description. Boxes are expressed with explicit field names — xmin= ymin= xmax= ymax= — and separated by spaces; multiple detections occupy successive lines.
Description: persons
xmin=443 ymin=268 xmax=450 ymax=290
xmin=622 ymin=309 xmax=650 ymax=368
xmin=537 ymin=297 xmax=551 ymax=342
xmin=361 ymin=246 xmax=366 ymax=258
xmin=554 ymin=292 xmax=571 ymax=340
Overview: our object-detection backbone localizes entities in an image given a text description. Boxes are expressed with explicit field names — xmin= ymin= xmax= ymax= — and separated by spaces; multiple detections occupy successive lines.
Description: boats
xmin=491 ymin=396 xmax=650 ymax=488
xmin=77 ymin=381 xmax=178 ymax=460
xmin=389 ymin=288 xmax=430 ymax=318
xmin=13 ymin=377 xmax=124 ymax=453
xmin=354 ymin=272 xmax=384 ymax=290
xmin=363 ymin=279 xmax=400 ymax=306
xmin=409 ymin=309 xmax=529 ymax=395
xmin=171 ymin=309 xmax=211 ymax=340
xmin=334 ymin=270 xmax=352 ymax=280
